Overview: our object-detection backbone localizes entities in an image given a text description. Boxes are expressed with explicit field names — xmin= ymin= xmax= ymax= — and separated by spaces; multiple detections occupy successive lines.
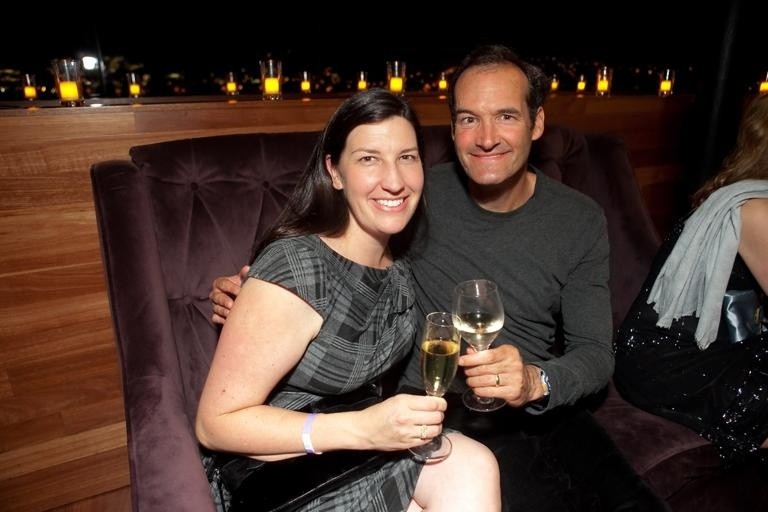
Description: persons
xmin=208 ymin=53 xmax=664 ymax=512
xmin=195 ymin=90 xmax=502 ymax=512
xmin=614 ymin=93 xmax=767 ymax=458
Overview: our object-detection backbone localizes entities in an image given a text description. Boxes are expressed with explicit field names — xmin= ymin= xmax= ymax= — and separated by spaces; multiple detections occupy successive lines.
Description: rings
xmin=496 ymin=374 xmax=500 ymax=387
xmin=421 ymin=425 xmax=428 ymax=439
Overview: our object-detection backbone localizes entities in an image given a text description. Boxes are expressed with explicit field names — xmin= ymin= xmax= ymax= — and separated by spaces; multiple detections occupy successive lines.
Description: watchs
xmin=540 ymin=369 xmax=551 ymax=395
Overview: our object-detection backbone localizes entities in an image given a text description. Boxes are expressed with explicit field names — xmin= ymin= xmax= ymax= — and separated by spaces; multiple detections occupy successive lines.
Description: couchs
xmin=90 ymin=124 xmax=768 ymax=511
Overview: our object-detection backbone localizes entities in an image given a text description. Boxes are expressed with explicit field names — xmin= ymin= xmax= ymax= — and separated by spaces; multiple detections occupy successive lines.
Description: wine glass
xmin=408 ymin=280 xmax=506 ymax=464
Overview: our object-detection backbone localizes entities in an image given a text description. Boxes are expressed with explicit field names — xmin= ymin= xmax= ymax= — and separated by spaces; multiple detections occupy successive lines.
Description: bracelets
xmin=302 ymin=412 xmax=323 ymax=455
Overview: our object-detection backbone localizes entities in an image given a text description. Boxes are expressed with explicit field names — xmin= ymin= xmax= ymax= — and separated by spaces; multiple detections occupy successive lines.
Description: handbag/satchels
xmin=717 ymin=289 xmax=768 ymax=345
xmin=207 ymin=381 xmax=408 ymax=512
